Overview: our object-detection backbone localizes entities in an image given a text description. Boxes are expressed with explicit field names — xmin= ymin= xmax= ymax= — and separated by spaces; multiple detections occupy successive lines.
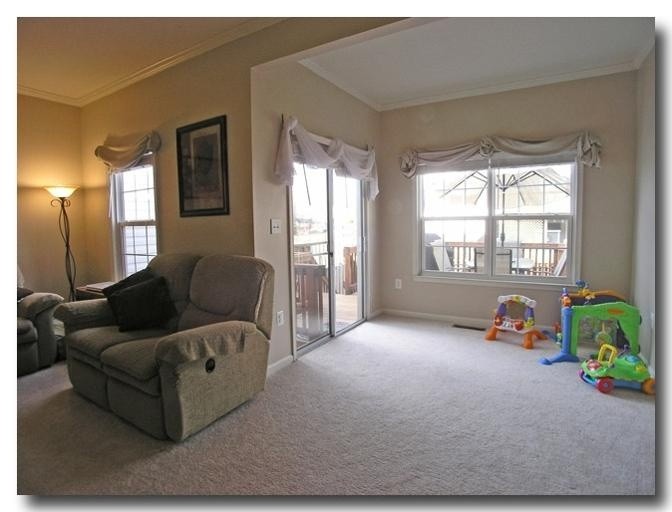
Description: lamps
xmin=44 ymin=186 xmax=80 ymax=303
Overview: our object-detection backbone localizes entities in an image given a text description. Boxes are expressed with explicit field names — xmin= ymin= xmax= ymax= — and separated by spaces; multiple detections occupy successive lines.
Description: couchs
xmin=53 ymin=253 xmax=275 ymax=442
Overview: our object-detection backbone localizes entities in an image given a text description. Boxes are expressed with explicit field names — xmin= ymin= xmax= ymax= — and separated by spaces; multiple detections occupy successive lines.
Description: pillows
xmin=109 ymin=276 xmax=177 ymax=332
xmin=17 ymin=286 xmax=33 ymax=303
xmin=102 ymin=268 xmax=166 ymax=329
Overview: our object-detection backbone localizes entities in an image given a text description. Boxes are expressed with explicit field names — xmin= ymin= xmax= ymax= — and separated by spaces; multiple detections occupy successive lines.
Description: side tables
xmin=76 ymin=287 xmax=105 ymax=301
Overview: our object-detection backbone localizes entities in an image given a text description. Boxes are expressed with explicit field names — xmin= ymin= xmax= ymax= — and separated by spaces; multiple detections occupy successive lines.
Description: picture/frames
xmin=176 ymin=114 xmax=230 ymax=217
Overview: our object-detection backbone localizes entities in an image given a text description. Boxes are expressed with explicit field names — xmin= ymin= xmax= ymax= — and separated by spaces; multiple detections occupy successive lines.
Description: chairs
xmin=431 ymin=245 xmax=568 ymax=276
xmin=17 ymin=265 xmax=64 ymax=378
